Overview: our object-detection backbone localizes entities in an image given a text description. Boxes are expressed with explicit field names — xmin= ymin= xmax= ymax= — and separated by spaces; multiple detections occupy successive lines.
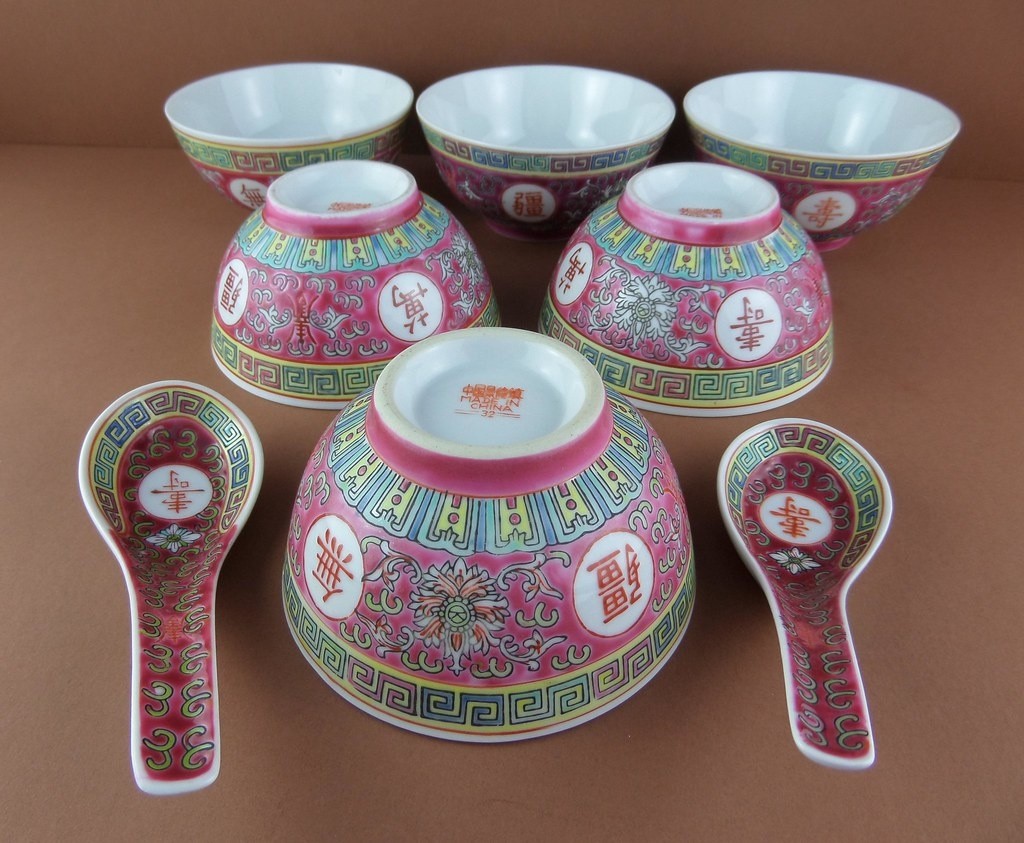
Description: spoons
xmin=715 ymin=419 xmax=895 ymax=774
xmin=78 ymin=379 xmax=267 ymax=795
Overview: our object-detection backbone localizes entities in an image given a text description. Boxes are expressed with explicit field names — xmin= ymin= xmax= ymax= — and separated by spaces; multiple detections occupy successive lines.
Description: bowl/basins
xmin=415 ymin=63 xmax=676 ymax=238
xmin=684 ymin=68 xmax=962 ymax=253
xmin=163 ymin=62 xmax=414 ymax=217
xmin=210 ymin=158 xmax=502 ymax=410
xmin=281 ymin=324 xmax=696 ymax=745
xmin=538 ymin=162 xmax=835 ymax=418
